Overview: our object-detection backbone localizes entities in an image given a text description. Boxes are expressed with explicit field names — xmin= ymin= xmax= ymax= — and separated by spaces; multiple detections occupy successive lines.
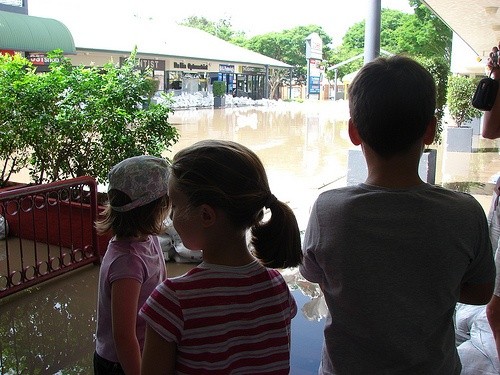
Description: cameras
xmin=491 ymin=51 xmax=500 ymax=69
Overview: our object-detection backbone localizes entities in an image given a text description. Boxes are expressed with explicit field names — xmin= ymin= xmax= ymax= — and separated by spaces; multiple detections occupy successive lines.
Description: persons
xmin=93 ymin=156 xmax=172 ymax=375
xmin=474 ymin=41 xmax=500 ymax=375
xmin=138 ymin=140 xmax=303 ymax=375
xmin=299 ymin=56 xmax=496 ymax=375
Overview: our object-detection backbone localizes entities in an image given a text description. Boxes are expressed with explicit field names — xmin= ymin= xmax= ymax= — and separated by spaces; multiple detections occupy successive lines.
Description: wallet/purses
xmin=472 ymin=67 xmax=497 ymax=110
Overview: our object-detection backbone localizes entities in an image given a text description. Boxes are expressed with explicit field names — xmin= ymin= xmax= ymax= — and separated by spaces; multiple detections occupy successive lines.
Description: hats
xmin=107 ymin=155 xmax=171 ymax=212
